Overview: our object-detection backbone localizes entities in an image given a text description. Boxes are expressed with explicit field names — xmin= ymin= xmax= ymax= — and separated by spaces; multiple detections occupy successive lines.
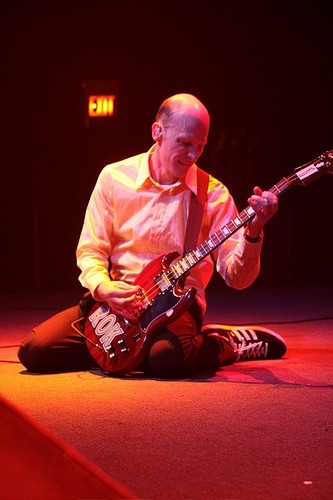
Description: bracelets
xmin=246 ymin=230 xmax=263 ymax=243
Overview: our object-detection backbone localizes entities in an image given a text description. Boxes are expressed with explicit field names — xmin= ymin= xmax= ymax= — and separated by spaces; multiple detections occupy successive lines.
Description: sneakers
xmin=202 ymin=324 xmax=287 ymax=362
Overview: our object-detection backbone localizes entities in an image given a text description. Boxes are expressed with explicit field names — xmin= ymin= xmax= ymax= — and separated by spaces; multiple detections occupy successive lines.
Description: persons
xmin=18 ymin=94 xmax=288 ymax=379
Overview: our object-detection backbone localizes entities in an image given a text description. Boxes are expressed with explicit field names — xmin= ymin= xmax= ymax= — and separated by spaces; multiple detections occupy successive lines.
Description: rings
xmin=263 ymin=200 xmax=269 ymax=206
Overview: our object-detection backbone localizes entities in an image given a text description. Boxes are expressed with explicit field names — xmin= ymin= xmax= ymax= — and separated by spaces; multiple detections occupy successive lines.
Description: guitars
xmin=82 ymin=152 xmax=333 ymax=379
